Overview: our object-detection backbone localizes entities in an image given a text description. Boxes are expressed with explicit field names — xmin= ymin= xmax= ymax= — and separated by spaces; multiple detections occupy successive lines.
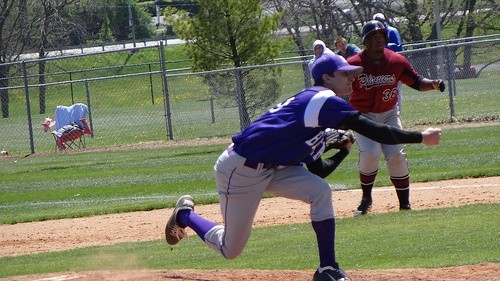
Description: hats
xmin=311 ymin=56 xmax=363 ymax=85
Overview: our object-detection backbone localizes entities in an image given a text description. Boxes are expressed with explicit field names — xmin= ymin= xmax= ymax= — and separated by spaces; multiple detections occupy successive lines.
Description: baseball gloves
xmin=324 ymin=128 xmax=356 ymax=153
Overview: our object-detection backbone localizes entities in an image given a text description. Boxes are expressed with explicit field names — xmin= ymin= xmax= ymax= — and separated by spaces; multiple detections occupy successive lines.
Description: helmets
xmin=361 ymin=20 xmax=388 ymax=45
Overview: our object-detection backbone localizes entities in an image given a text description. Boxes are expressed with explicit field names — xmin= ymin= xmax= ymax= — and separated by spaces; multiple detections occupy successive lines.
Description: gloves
xmin=432 ymin=80 xmax=445 ymax=91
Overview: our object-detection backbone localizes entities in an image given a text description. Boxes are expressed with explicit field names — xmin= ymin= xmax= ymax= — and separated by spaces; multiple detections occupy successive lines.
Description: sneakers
xmin=313 ymin=265 xmax=348 ymax=281
xmin=166 ymin=194 xmax=196 ymax=245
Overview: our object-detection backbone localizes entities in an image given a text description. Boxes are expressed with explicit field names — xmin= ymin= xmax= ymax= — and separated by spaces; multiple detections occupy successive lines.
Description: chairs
xmin=41 ymin=102 xmax=94 ymax=155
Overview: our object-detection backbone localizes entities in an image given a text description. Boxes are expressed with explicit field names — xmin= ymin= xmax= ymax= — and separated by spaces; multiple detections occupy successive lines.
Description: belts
xmin=228 ymin=148 xmax=271 ymax=170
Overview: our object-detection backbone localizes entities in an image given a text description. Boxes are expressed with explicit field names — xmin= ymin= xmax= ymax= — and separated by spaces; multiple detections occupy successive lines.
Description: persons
xmin=347 ymin=20 xmax=446 ymax=217
xmin=164 ymin=55 xmax=441 ymax=281
xmin=308 ymin=40 xmax=333 ymax=68
xmin=334 ymin=36 xmax=360 ymax=58
xmin=373 ymin=13 xmax=403 ymax=114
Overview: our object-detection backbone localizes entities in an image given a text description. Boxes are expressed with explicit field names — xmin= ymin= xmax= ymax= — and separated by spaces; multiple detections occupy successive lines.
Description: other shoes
xmin=400 ymin=205 xmax=412 ymax=211
xmin=354 ymin=200 xmax=372 ymax=217
